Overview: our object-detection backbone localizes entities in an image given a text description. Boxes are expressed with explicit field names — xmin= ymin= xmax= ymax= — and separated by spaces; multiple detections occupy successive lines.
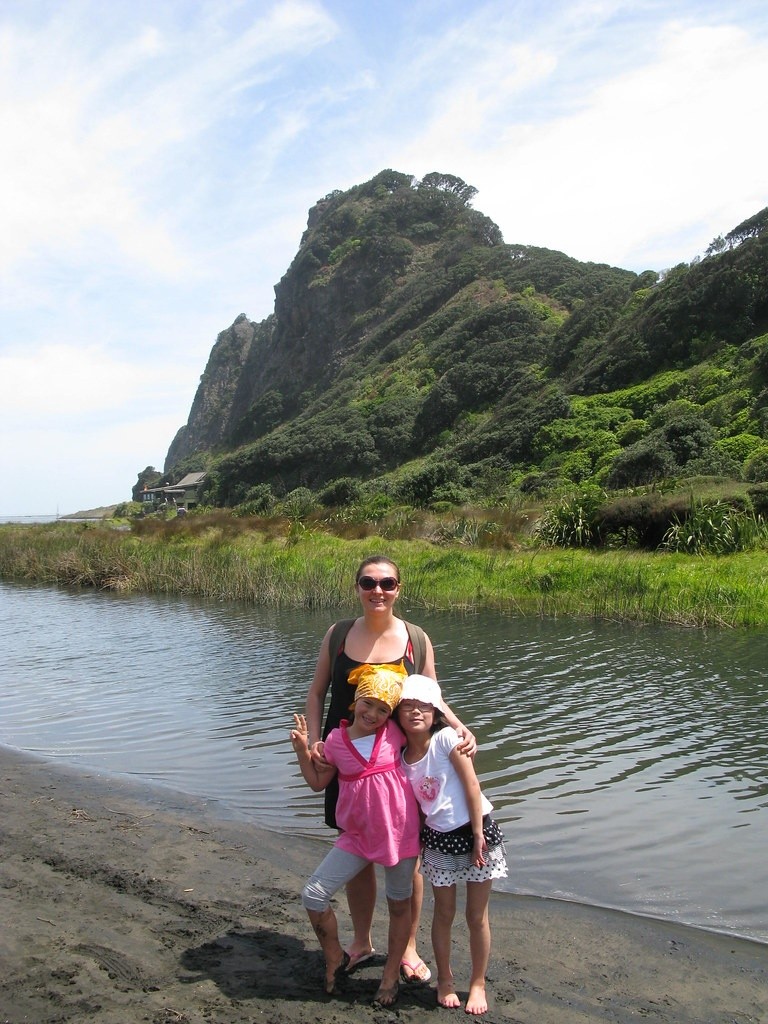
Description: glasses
xmin=401 ymin=702 xmax=437 ymax=711
xmin=359 ymin=575 xmax=400 ymax=591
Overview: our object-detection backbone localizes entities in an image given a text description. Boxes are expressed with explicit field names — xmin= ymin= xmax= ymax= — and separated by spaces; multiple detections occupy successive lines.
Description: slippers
xmin=401 ymin=958 xmax=431 ymax=984
xmin=345 ymin=944 xmax=376 ymax=971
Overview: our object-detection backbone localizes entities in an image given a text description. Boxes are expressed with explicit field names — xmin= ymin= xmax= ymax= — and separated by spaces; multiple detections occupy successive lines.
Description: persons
xmin=304 ymin=555 xmax=478 ymax=984
xmin=290 ymin=659 xmax=509 ymax=1015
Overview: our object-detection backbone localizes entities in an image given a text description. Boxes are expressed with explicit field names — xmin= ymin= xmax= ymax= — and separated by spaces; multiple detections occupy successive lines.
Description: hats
xmin=399 ymin=674 xmax=444 ymax=719
